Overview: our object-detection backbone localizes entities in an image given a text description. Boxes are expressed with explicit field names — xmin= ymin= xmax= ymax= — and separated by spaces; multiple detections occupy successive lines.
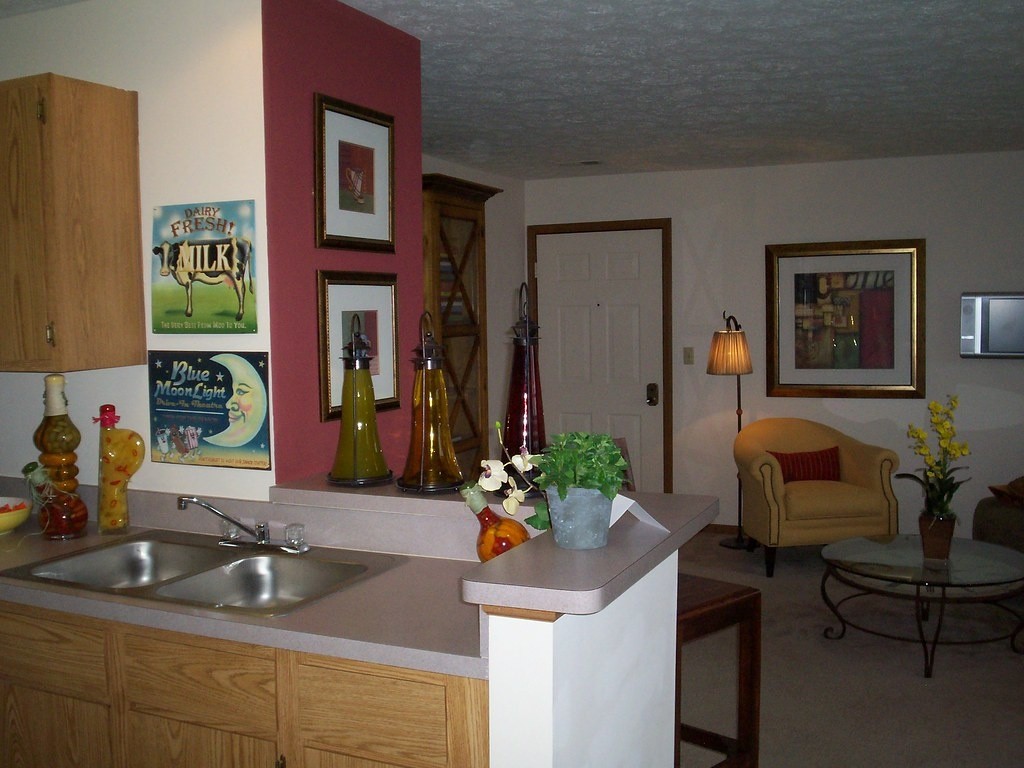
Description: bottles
xmin=330 ymin=333 xmax=388 ymax=480
xmin=459 ymin=481 xmax=531 ymax=564
xmin=34 ymin=374 xmax=90 ymax=540
xmin=500 ymin=337 xmax=545 ymax=492
xmin=401 ymin=335 xmax=463 ymax=487
xmin=98 ymin=404 xmax=145 ymax=536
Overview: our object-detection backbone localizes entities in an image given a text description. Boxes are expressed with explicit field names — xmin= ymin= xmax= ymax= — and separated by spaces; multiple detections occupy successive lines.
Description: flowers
xmin=892 ymin=393 xmax=974 ymax=516
xmin=478 ymin=422 xmax=542 ymax=514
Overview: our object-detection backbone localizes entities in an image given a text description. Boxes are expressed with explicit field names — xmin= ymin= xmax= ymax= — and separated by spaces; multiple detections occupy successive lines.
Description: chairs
xmin=612 ymin=435 xmax=761 ymax=768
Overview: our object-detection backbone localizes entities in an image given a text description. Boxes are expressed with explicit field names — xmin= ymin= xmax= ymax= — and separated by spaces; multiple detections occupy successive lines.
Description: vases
xmin=919 ymin=506 xmax=957 ymax=560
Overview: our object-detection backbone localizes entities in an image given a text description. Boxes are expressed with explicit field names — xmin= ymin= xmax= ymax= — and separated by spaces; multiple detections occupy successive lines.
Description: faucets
xmin=176 ymin=494 xmax=271 ymax=545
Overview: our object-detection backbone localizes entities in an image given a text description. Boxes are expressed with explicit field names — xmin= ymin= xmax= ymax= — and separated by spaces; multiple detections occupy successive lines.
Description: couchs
xmin=973 ymin=474 xmax=1024 ymax=550
xmin=733 ymin=418 xmax=899 ymax=577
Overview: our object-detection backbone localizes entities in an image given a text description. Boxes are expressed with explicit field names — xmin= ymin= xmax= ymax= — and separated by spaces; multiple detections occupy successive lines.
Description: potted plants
xmin=523 ymin=431 xmax=628 ymax=552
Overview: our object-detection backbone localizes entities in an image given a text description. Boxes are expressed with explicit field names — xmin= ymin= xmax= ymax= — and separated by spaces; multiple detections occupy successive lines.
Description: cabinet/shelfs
xmin=0 ymin=597 xmax=489 ymax=768
xmin=1 ymin=72 xmax=147 ymax=372
xmin=421 ymin=172 xmax=504 ymax=486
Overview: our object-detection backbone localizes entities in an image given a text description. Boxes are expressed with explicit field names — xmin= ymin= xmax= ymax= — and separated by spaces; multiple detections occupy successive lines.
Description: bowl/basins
xmin=0 ymin=496 xmax=33 ymax=535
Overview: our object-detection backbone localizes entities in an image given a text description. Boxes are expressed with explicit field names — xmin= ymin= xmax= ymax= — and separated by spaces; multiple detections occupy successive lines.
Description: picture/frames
xmin=313 ymin=91 xmax=396 ymax=253
xmin=765 ymin=238 xmax=926 ymax=400
xmin=318 ymin=269 xmax=401 ymax=424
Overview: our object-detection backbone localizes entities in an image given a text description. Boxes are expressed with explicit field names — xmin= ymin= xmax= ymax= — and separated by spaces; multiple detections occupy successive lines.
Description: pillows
xmin=766 ymin=447 xmax=840 ymax=483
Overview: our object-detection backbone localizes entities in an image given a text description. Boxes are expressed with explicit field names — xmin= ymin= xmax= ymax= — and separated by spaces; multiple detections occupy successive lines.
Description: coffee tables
xmin=821 ymin=534 xmax=1024 ymax=679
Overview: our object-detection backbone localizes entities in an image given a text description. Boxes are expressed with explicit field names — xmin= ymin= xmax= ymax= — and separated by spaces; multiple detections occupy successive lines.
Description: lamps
xmin=705 ymin=310 xmax=760 ymax=550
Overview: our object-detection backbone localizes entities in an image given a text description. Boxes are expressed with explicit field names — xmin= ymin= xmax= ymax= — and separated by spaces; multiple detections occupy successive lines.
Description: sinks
xmin=25 ymin=539 xmax=233 ymax=589
xmin=153 ymin=555 xmax=367 ymax=608
